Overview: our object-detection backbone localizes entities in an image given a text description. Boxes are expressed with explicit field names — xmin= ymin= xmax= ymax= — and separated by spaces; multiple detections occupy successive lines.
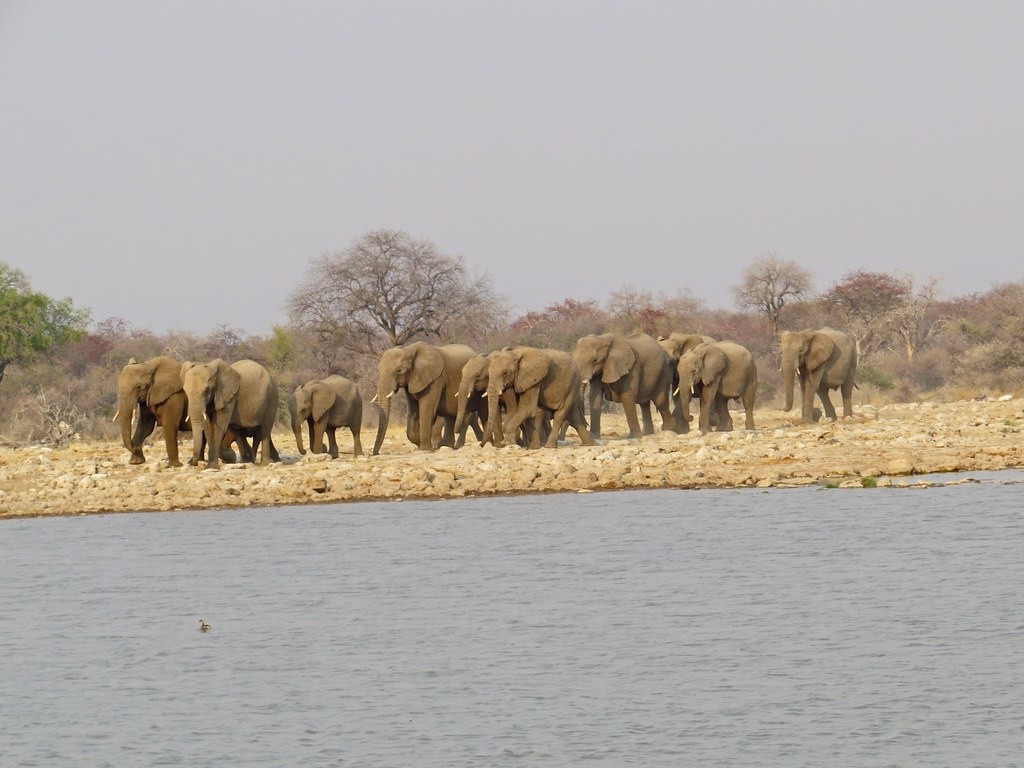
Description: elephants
xmin=111 ymin=324 xmax=860 ymax=470
